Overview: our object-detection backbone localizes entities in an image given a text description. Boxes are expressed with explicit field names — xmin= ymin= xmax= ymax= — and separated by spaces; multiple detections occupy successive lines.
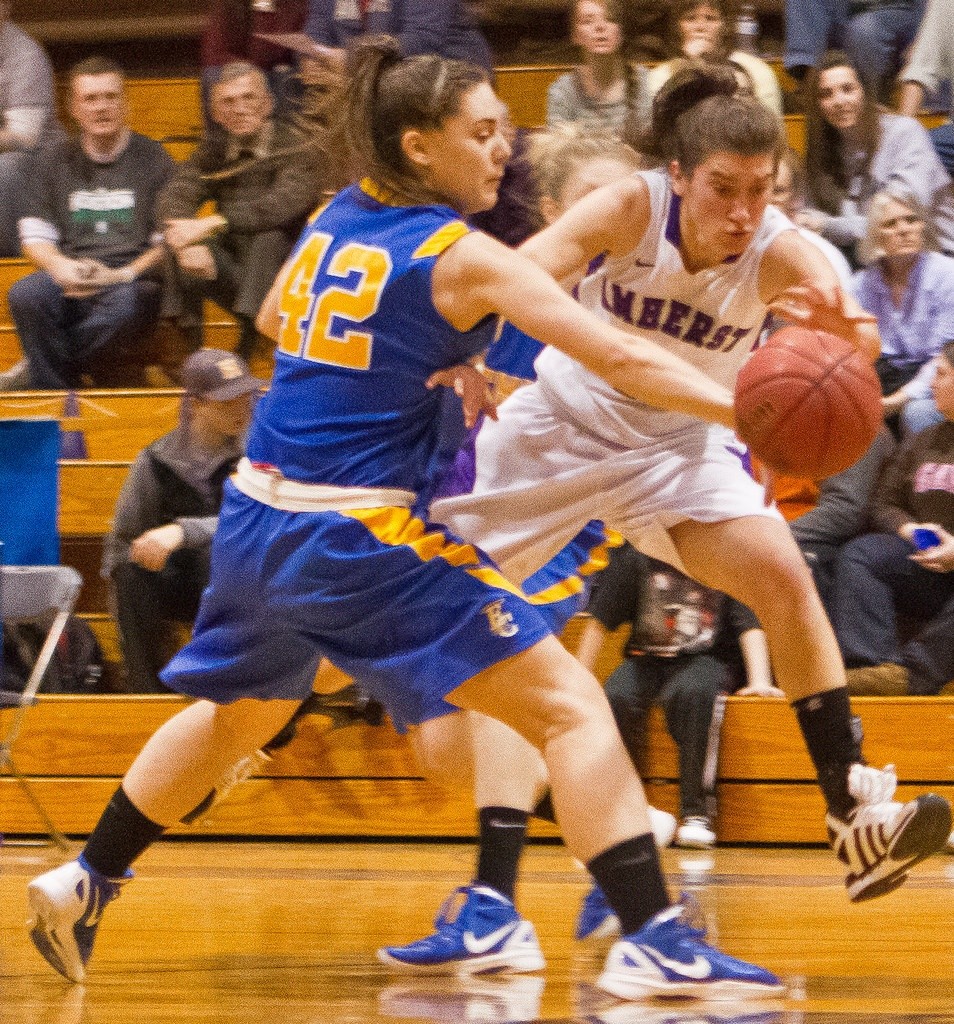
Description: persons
xmin=179 ymin=71 xmax=953 ymax=902
xmin=0 ymin=0 xmax=954 ymax=971
xmin=27 ymin=34 xmax=791 ymax=997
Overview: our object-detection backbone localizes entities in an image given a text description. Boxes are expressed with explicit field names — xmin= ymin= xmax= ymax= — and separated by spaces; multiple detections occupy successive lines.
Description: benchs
xmin=0 ymin=66 xmax=954 ymax=845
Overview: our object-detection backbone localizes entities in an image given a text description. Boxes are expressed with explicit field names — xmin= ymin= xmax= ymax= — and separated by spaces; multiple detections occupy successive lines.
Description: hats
xmin=187 ymin=347 xmax=260 ymax=400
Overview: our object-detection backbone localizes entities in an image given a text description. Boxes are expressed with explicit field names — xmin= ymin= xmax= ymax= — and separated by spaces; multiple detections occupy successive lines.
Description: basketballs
xmin=734 ymin=325 xmax=885 ymax=482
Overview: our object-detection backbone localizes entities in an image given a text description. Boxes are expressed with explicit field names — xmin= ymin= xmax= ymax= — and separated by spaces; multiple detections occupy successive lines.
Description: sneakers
xmin=596 ymin=905 xmax=782 ymax=1003
xmin=26 ymin=855 xmax=134 ymax=982
xmin=376 ymin=884 xmax=547 ymax=976
xmin=676 ymin=812 xmax=716 ymax=848
xmin=576 ymin=805 xmax=675 ymax=942
xmin=822 ymin=761 xmax=954 ymax=902
